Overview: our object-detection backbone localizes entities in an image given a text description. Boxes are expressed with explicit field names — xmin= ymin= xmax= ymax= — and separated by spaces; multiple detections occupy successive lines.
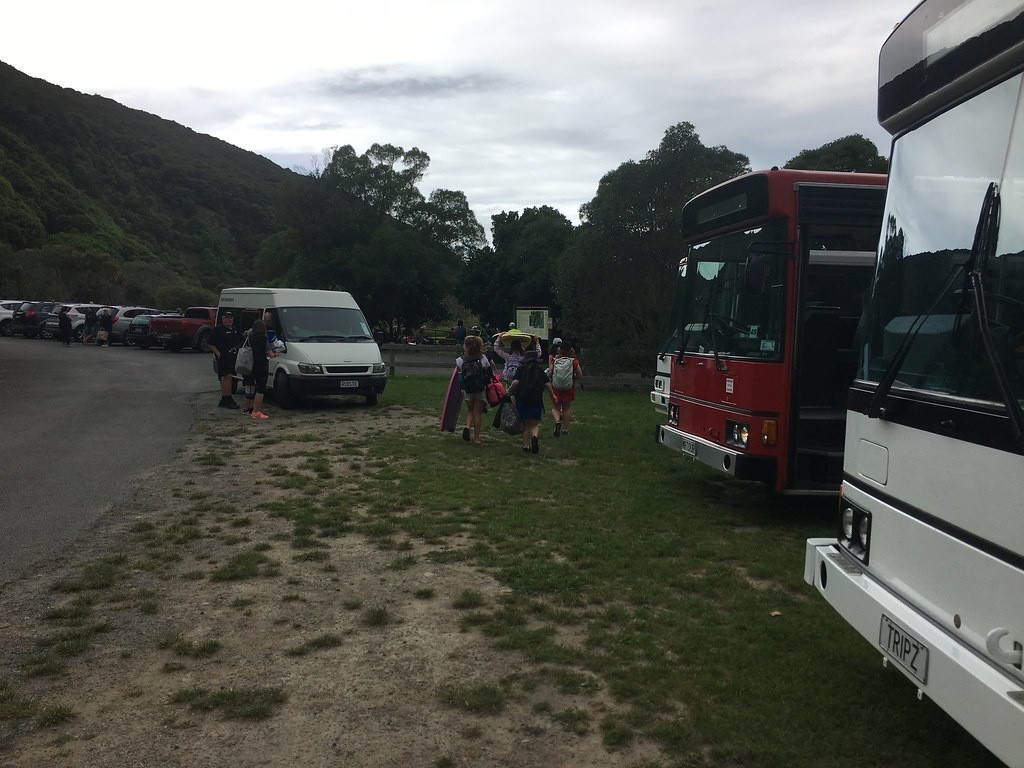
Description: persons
xmin=206 ymin=311 xmax=240 ymax=408
xmin=456 ymin=335 xmax=497 ymax=443
xmin=82 ymin=308 xmax=113 ymax=348
xmin=59 ymin=306 xmax=75 ymax=347
xmin=247 ymin=312 xmax=280 ymax=420
xmin=494 ymin=331 xmax=542 ymax=401
xmin=402 ymin=325 xmax=426 ymax=337
xmin=454 ymin=320 xmax=480 ymax=340
xmin=509 ymin=322 xmax=515 ymax=330
xmin=548 ymin=337 xmax=583 ymax=436
xmin=504 ymin=343 xmax=559 ymax=455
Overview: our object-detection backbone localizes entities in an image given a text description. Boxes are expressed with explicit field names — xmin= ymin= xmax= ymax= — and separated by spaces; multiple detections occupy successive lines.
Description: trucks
xmin=802 ymin=1 xmax=1024 ymax=768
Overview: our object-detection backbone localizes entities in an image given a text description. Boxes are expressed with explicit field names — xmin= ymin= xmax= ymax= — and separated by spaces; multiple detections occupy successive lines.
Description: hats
xmin=553 ymin=338 xmax=562 ymax=345
xmin=520 ymin=351 xmax=543 ymax=365
xmin=223 ymin=312 xmax=233 ymax=317
xmin=509 ymin=322 xmax=515 ymax=326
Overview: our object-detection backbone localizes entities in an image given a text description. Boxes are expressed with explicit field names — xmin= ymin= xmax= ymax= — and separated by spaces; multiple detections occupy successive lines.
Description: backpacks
xmin=550 ymin=357 xmax=576 ymax=391
xmin=461 ymin=356 xmax=485 ymax=394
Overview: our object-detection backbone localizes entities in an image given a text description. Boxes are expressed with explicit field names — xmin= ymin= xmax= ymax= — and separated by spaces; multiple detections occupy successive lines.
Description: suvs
xmin=0 ymin=300 xmax=63 ymax=338
xmin=96 ymin=305 xmax=158 ymax=346
xmin=45 ymin=302 xmax=105 ymax=342
xmin=125 ymin=309 xmax=165 ymax=350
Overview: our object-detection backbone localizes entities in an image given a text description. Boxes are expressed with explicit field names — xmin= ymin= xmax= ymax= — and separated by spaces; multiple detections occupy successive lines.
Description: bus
xmin=651 ymin=165 xmax=1024 ymax=509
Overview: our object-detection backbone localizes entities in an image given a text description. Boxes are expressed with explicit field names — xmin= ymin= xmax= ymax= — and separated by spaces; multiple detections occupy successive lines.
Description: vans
xmin=212 ymin=286 xmax=387 ymax=411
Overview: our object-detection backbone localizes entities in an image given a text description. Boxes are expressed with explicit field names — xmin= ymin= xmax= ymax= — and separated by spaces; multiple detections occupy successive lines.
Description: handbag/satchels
xmin=487 ymin=375 xmax=508 ymax=407
xmin=499 ymin=402 xmax=523 ymax=436
xmin=97 ymin=327 xmax=108 ymax=341
xmin=235 ymin=336 xmax=253 ymax=376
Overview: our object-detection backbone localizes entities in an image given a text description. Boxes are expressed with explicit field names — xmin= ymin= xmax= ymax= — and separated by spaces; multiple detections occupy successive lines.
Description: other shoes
xmin=462 ymin=427 xmax=471 ymax=441
xmin=218 ymin=397 xmax=240 ymax=409
xmin=561 ymin=430 xmax=569 ymax=438
xmin=102 ymin=344 xmax=108 ymax=347
xmin=553 ymin=422 xmax=561 ymax=437
xmin=531 ymin=435 xmax=539 ymax=454
xmin=523 ymin=445 xmax=530 ymax=453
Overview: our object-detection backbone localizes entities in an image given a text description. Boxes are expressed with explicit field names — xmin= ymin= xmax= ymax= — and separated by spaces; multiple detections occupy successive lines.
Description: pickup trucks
xmin=150 ymin=306 xmax=218 ymax=354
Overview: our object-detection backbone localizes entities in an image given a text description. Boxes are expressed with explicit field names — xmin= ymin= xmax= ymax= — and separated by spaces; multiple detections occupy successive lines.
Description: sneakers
xmin=242 ymin=408 xmax=253 ymax=415
xmin=251 ymin=411 xmax=269 ymax=419
